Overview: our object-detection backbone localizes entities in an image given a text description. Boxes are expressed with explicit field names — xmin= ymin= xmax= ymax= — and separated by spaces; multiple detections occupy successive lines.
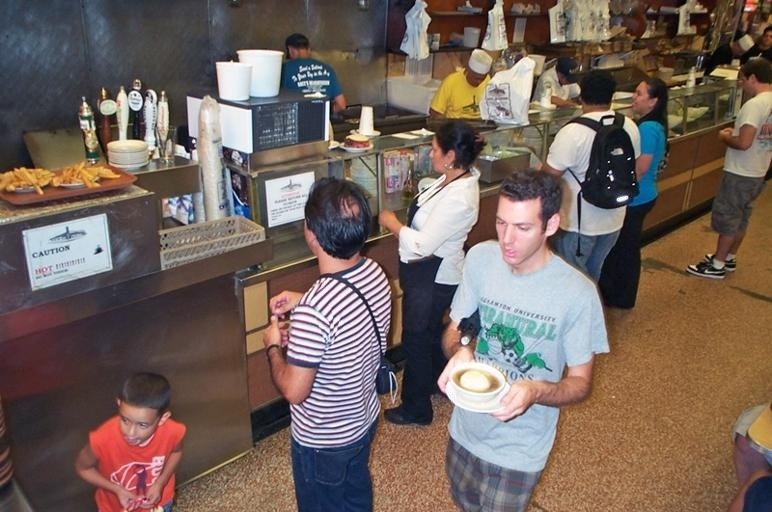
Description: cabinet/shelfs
xmin=529 ymin=86 xmax=740 ymax=248
xmin=387 ymin=1 xmax=717 ymax=84
xmin=227 ymin=113 xmax=548 ymax=439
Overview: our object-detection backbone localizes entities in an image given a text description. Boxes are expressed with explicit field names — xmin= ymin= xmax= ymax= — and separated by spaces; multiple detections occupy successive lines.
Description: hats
xmin=557 ymin=57 xmax=581 ymax=83
xmin=739 ymin=34 xmax=755 ymax=52
xmin=468 ymin=48 xmax=493 ymax=75
xmin=285 ymin=33 xmax=308 ymax=59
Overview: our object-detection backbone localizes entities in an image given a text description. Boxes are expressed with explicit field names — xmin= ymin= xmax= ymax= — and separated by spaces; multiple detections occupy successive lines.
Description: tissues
xmin=560 ymin=83 xmax=581 ymax=100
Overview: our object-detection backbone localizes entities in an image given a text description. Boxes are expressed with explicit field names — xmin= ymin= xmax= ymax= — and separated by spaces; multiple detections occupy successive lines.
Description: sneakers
xmin=704 ymin=253 xmax=737 ymax=272
xmin=686 ymin=260 xmax=725 ymax=280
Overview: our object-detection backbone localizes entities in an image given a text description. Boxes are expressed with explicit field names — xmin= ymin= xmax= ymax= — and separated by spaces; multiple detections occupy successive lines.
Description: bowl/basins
xmin=666 ymin=114 xmax=683 ymax=130
xmin=678 ymin=108 xmax=708 ymax=122
xmin=450 ymin=363 xmax=505 ymax=401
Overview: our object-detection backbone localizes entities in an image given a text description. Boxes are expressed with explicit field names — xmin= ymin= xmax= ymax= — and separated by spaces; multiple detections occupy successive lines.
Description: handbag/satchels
xmin=376 ymin=357 xmax=400 ymax=405
xmin=656 ymin=140 xmax=671 ymax=180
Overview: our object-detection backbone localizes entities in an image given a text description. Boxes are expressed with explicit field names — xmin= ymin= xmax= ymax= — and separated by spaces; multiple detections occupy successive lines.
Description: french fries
xmin=0 ymin=166 xmax=56 ymax=196
xmin=50 ymin=160 xmax=121 ymax=189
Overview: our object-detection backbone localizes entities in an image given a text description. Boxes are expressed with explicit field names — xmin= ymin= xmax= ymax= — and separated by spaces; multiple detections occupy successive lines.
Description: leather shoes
xmin=384 ymin=407 xmax=433 ymax=425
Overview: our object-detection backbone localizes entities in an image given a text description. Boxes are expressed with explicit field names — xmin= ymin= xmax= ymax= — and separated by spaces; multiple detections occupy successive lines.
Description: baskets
xmin=160 ymin=215 xmax=266 ymax=272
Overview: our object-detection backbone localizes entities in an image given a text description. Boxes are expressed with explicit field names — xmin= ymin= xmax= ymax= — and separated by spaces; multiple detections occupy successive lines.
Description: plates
xmin=351 ymin=156 xmax=379 ymax=219
xmin=522 ymin=128 xmax=545 ymax=140
xmin=328 ymin=141 xmax=340 ymax=151
xmin=444 ymin=378 xmax=512 ymax=411
xmin=59 ymin=177 xmax=99 ymax=191
xmin=339 ymin=143 xmax=374 ymax=153
xmin=349 ymin=130 xmax=381 ymax=139
xmin=15 ymin=181 xmax=49 ymax=194
xmin=107 ymin=139 xmax=151 ymax=171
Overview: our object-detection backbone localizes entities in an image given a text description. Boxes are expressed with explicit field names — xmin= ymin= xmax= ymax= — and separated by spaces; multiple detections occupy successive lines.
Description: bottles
xmin=686 ymin=68 xmax=696 ymax=87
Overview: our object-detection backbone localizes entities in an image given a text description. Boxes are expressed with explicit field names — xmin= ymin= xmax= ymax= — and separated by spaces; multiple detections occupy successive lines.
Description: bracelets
xmin=264 ymin=344 xmax=281 ymax=354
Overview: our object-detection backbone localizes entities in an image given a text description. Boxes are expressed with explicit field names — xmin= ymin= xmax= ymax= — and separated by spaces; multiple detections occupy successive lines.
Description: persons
xmin=427 ymin=64 xmax=493 ymax=123
xmin=280 ymin=33 xmax=347 ymax=118
xmin=72 ymin=371 xmax=187 ymax=512
xmin=684 ymin=59 xmax=772 ymax=279
xmin=742 ymin=26 xmax=772 ymax=64
xmin=377 ymin=120 xmax=486 ymax=424
xmin=437 ymin=168 xmax=610 ymax=512
xmin=535 ymin=68 xmax=639 ymax=287
xmin=720 ymin=399 xmax=772 ymax=512
xmin=263 ymin=178 xmax=392 ymax=511
xmin=600 ymin=77 xmax=668 ymax=306
xmin=698 ymin=41 xmax=750 ymax=80
xmin=532 ymin=57 xmax=581 ymax=108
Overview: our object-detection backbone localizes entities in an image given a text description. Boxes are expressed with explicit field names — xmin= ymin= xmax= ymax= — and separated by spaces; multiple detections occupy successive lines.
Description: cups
xmin=463 ymin=27 xmax=481 ymax=49
xmin=194 ymin=105 xmax=231 ymax=224
xmin=360 ymin=106 xmax=375 ymax=136
xmin=155 ymin=125 xmax=178 ymax=166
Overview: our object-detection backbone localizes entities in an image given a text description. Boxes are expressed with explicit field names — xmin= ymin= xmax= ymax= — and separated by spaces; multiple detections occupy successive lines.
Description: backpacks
xmin=561 ymin=111 xmax=641 ymax=257
